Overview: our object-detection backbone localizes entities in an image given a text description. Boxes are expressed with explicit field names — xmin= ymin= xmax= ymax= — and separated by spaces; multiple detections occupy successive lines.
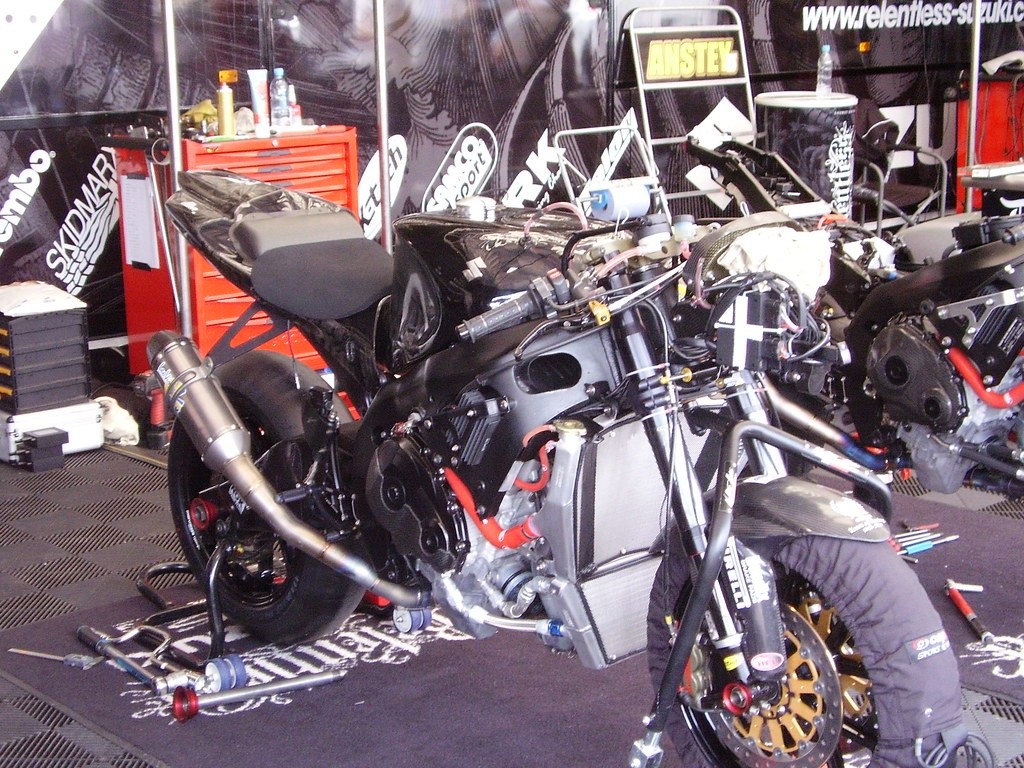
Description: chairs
xmin=855 ymin=99 xmax=947 ymax=238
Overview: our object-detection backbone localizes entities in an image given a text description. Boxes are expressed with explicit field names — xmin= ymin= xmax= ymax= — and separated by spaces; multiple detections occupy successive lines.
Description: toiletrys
xmin=246 ymin=67 xmax=270 ymax=138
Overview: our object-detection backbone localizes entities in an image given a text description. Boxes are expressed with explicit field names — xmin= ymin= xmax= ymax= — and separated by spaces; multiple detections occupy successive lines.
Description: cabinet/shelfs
xmin=114 ymin=123 xmax=360 ymax=414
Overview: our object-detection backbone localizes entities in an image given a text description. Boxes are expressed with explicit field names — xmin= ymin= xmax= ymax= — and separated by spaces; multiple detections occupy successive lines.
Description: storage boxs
xmin=0 ymin=283 xmax=92 ymax=418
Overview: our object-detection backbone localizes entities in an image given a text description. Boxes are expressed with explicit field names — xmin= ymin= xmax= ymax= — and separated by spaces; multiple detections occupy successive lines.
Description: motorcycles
xmin=133 ymin=2 xmax=1024 ymax=768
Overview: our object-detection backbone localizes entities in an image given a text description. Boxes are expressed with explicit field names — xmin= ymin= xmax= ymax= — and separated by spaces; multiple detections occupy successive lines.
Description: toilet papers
xmin=590 ymin=183 xmax=651 ymax=219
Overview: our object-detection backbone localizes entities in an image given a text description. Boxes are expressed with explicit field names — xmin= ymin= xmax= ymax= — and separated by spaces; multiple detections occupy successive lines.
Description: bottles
xmin=270 ymin=67 xmax=289 ymax=133
xmin=817 ymin=45 xmax=833 ymax=100
xmin=216 ymin=83 xmax=234 ymax=135
